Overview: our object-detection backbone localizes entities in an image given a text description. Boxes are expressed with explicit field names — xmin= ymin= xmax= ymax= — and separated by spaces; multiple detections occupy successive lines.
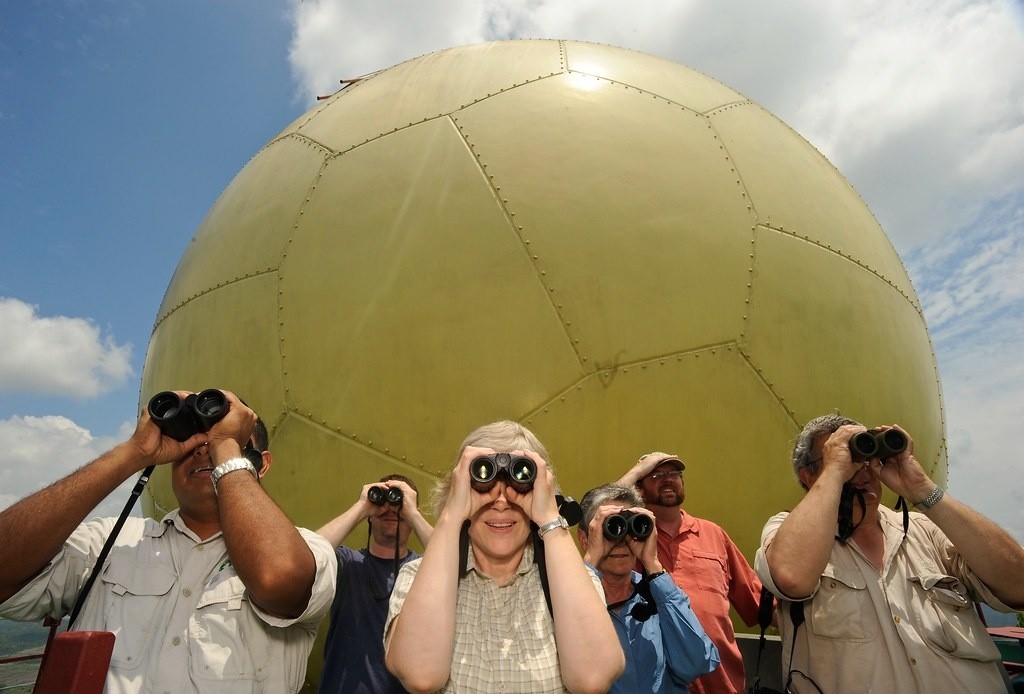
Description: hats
xmin=637 ymin=454 xmax=686 ymax=472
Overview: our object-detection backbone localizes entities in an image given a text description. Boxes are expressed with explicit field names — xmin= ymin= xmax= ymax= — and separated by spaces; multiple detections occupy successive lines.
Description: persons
xmin=314 ymin=475 xmax=441 ymax=694
xmin=380 ymin=417 xmax=628 ymax=694
xmin=755 ymin=413 xmax=1024 ymax=694
xmin=579 ymin=450 xmax=783 ymax=694
xmin=0 ymin=387 xmax=338 ymax=694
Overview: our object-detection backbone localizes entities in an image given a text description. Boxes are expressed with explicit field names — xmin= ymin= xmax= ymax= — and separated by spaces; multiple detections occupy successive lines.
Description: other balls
xmin=133 ymin=37 xmax=950 ymax=638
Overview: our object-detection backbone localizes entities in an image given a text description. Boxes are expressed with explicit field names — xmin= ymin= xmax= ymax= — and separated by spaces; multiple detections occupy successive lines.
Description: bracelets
xmin=209 ymin=457 xmax=258 ymax=495
xmin=537 ymin=515 xmax=569 ymax=539
xmin=912 ymin=484 xmax=944 ymax=513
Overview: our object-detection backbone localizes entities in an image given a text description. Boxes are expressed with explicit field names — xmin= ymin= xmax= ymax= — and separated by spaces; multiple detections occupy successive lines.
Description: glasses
xmin=644 ymin=470 xmax=682 ymax=480
